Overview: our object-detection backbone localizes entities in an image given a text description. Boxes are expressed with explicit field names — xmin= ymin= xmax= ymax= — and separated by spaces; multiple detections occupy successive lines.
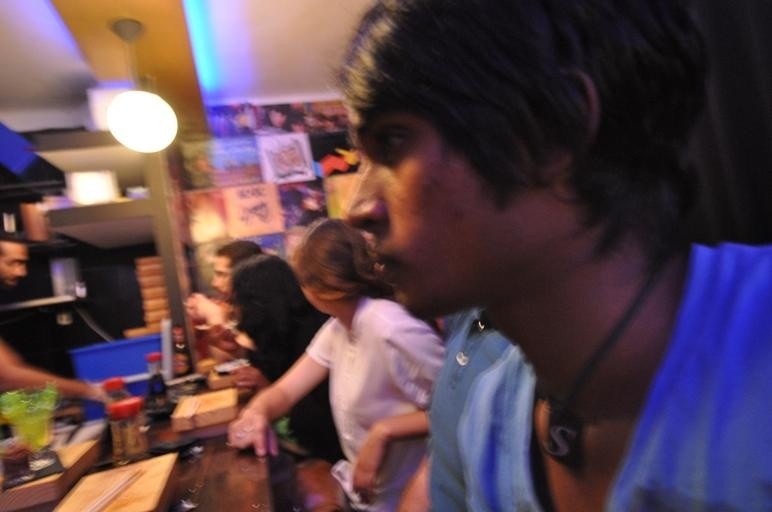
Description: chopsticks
xmin=186 ymin=398 xmax=202 ymax=420
xmin=85 ymin=469 xmax=144 ymax=511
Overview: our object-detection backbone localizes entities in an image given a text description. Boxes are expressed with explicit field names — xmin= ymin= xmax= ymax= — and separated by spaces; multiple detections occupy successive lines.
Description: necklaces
xmin=535 ymin=246 xmax=665 ymax=462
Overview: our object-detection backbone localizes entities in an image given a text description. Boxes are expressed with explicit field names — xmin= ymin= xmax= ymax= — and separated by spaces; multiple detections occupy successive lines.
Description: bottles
xmin=146 ymin=352 xmax=170 ymax=415
xmin=172 ymin=326 xmax=189 ymax=374
xmin=101 ymin=375 xmax=132 ymax=404
xmin=3 ymin=438 xmax=30 ymax=483
xmin=109 ymin=398 xmax=147 ymax=464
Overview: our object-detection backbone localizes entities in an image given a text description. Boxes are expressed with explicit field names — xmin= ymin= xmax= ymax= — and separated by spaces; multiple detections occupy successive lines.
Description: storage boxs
xmin=66 ymin=332 xmax=163 ymax=421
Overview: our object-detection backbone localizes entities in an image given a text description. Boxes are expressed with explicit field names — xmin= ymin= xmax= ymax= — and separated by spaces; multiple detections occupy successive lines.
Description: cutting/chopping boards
xmin=171 ymin=387 xmax=239 ymax=432
xmin=53 ymin=451 xmax=178 ymax=512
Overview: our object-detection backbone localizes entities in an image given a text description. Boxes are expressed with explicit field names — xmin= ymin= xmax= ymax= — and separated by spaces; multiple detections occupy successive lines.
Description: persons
xmin=254 ymin=104 xmax=301 ymax=133
xmin=274 ymin=145 xmax=306 ymax=178
xmin=3 ymin=237 xmax=120 ymax=407
xmin=338 ymin=0 xmax=771 ymax=511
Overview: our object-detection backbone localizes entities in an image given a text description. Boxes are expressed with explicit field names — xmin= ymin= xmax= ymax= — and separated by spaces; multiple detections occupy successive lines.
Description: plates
xmin=212 ymin=359 xmax=250 ymax=373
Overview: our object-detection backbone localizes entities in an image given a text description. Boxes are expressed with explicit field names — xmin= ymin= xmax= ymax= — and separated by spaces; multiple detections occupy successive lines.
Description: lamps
xmin=105 ymin=14 xmax=179 ymax=159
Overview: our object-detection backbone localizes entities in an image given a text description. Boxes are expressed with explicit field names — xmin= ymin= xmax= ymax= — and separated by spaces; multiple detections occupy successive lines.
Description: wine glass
xmin=3 ymin=389 xmax=61 ymax=468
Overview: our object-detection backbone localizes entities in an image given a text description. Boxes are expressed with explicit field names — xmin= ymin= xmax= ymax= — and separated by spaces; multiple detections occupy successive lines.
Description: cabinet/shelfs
xmin=28 ymin=126 xmax=154 ymax=250
